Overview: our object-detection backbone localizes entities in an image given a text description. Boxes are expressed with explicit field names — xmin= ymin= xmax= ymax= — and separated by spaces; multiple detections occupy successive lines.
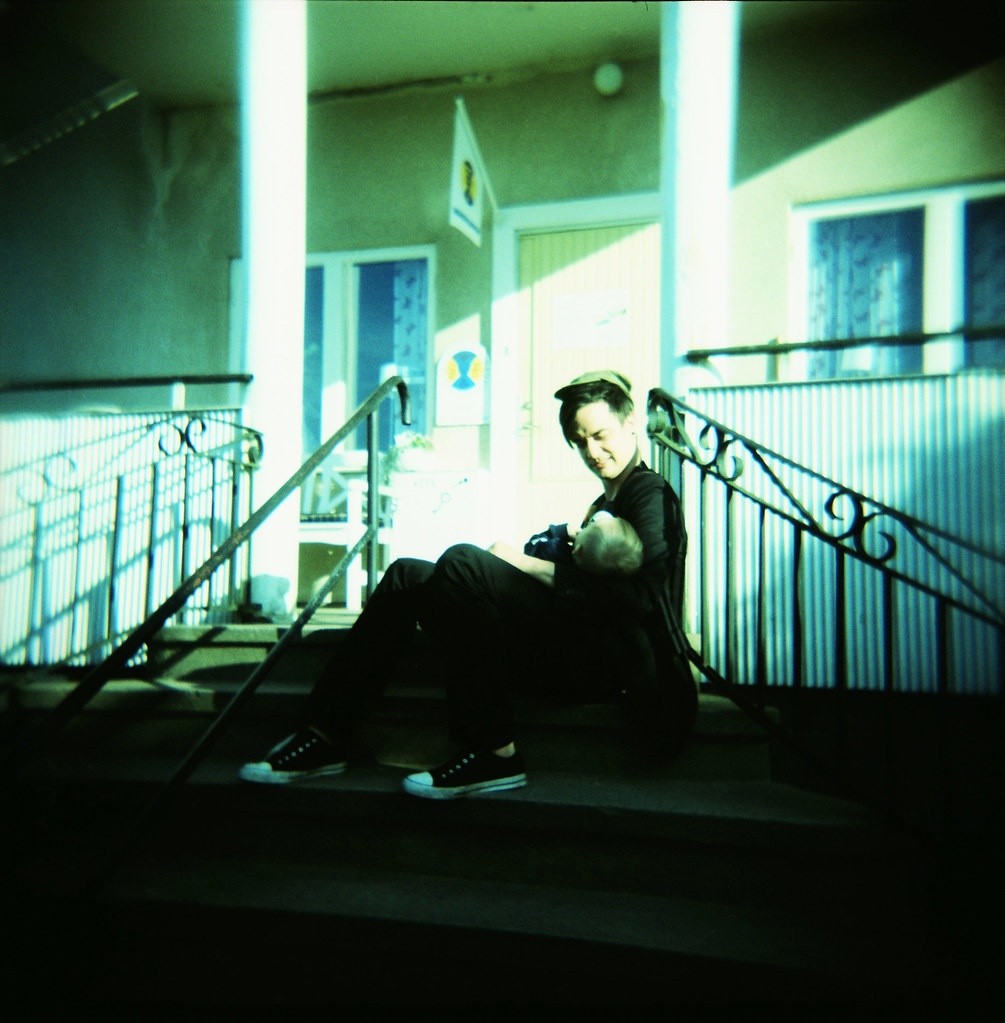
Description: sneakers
xmin=402 ymin=746 xmax=528 ymax=801
xmin=239 ymin=729 xmax=346 ymax=784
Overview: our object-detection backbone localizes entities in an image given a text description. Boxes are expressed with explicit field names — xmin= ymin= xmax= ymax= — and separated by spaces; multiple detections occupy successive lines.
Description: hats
xmin=555 ymin=370 xmax=634 ymax=409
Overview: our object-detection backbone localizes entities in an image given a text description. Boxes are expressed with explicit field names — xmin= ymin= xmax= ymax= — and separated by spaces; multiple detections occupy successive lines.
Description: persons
xmin=239 ymin=371 xmax=699 ymax=799
